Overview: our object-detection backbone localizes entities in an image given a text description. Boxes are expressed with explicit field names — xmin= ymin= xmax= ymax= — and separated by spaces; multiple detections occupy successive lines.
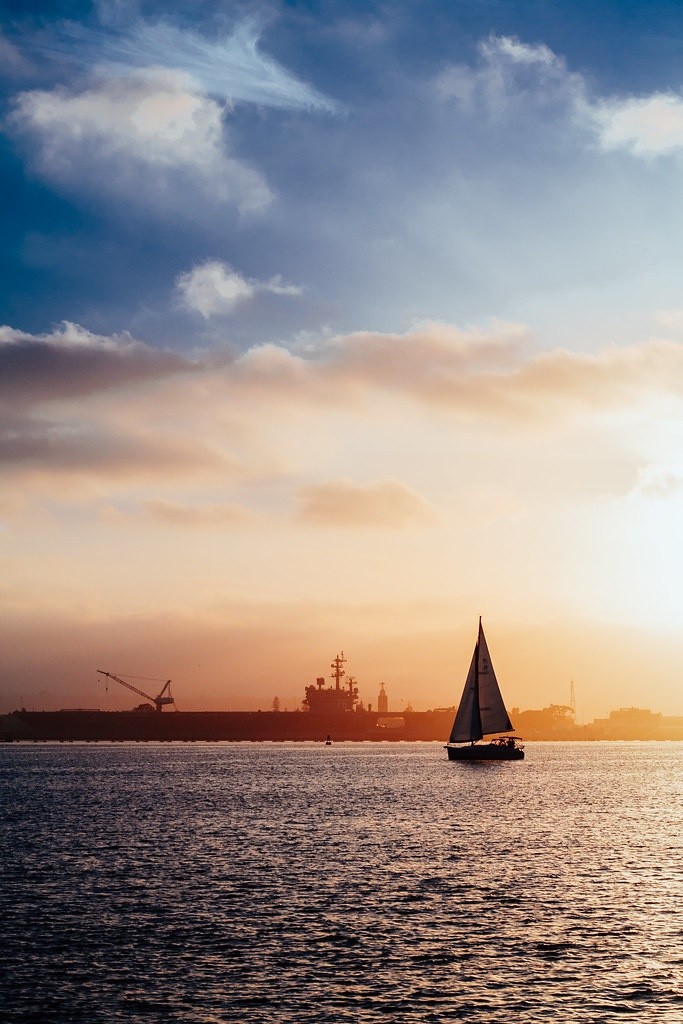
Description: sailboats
xmin=443 ymin=615 xmax=525 ymax=759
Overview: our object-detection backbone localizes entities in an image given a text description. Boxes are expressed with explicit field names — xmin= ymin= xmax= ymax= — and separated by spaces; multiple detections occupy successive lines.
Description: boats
xmin=300 ymin=651 xmax=360 ymax=711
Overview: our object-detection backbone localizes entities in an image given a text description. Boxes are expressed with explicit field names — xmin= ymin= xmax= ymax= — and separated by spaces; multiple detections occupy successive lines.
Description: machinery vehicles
xmin=95 ymin=668 xmax=175 ymax=712
